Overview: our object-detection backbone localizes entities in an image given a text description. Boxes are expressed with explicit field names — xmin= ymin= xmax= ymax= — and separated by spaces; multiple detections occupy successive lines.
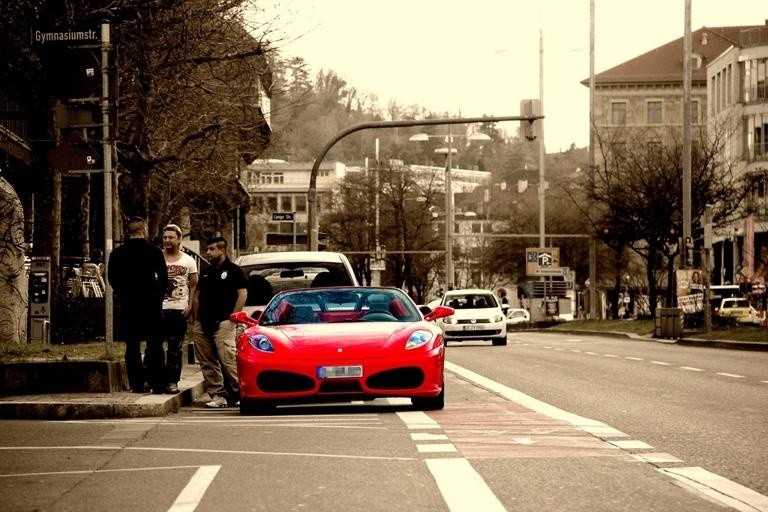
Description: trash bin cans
xmin=658 ymin=308 xmax=683 ymax=340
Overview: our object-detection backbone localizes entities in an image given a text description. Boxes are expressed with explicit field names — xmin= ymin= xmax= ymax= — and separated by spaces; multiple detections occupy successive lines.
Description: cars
xmin=713 ymin=296 xmax=755 ymax=324
xmin=504 ymin=307 xmax=531 ymax=325
xmin=438 ymin=287 xmax=513 ymax=349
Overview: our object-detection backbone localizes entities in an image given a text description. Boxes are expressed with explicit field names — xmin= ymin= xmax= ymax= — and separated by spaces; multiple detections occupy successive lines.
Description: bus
xmin=689 ymin=284 xmax=768 ymax=326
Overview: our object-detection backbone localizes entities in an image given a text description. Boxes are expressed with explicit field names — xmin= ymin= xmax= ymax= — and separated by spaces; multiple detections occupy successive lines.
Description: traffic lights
xmin=317 ymin=232 xmax=329 ymax=250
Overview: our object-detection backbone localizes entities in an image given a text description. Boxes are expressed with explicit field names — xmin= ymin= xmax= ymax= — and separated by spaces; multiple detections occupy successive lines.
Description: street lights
xmin=363 ymin=130 xmax=496 ymax=289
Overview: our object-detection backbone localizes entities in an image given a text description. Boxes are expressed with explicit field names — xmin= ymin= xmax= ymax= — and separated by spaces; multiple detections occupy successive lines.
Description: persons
xmin=190 ymin=236 xmax=248 ymax=409
xmin=605 ymin=291 xmax=644 ymax=322
xmin=654 ymin=254 xmax=669 ymax=290
xmin=688 ymin=270 xmax=701 ymax=294
xmin=141 ymin=223 xmax=199 ymax=395
xmin=108 ymin=215 xmax=168 ymax=393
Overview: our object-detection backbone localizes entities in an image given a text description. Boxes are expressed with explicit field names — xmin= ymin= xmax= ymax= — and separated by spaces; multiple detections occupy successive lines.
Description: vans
xmin=226 ymin=248 xmax=359 ymax=323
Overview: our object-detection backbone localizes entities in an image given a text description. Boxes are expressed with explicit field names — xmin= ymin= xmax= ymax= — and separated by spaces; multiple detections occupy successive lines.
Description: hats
xmin=163 ymin=224 xmax=183 ymax=250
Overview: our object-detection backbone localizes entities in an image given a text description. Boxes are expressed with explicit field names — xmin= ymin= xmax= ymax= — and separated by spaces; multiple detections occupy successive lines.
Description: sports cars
xmin=222 ymin=285 xmax=463 ymax=415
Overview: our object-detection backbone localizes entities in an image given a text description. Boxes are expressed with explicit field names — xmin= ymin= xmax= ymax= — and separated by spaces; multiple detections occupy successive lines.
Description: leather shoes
xmin=205 ymin=395 xmax=240 ymax=408
xmin=130 ymin=382 xmax=180 ymax=394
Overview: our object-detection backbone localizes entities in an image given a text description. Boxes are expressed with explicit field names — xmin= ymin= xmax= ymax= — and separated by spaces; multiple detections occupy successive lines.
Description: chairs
xmin=285 ymin=306 xmax=314 ymax=323
xmin=370 ymin=303 xmax=388 ymax=312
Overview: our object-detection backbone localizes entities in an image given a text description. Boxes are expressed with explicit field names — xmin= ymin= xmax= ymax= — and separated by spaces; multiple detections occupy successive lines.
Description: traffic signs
xmin=31 ymin=22 xmax=102 ymax=49
xmin=272 ymin=212 xmax=294 ymax=220
